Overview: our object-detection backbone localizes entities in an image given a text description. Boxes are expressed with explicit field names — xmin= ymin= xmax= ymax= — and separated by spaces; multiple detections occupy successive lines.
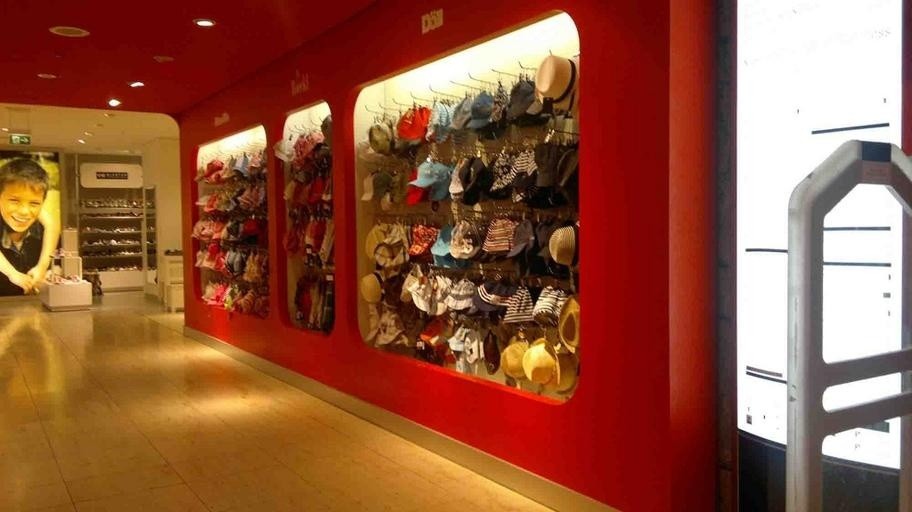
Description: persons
xmin=0 ymin=195 xmax=59 ymax=295
xmin=1 ymin=158 xmax=61 ymax=297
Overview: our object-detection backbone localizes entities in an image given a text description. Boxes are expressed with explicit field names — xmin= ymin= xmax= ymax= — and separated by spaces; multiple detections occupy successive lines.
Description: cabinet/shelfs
xmin=79 ymin=207 xmax=156 ymax=259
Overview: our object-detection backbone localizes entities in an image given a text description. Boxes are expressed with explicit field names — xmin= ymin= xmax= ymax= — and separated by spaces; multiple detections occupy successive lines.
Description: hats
xmin=275 ymin=117 xmax=338 ymax=268
xmin=360 ymin=54 xmax=581 ymax=390
xmin=194 ymin=116 xmax=270 ymax=316
